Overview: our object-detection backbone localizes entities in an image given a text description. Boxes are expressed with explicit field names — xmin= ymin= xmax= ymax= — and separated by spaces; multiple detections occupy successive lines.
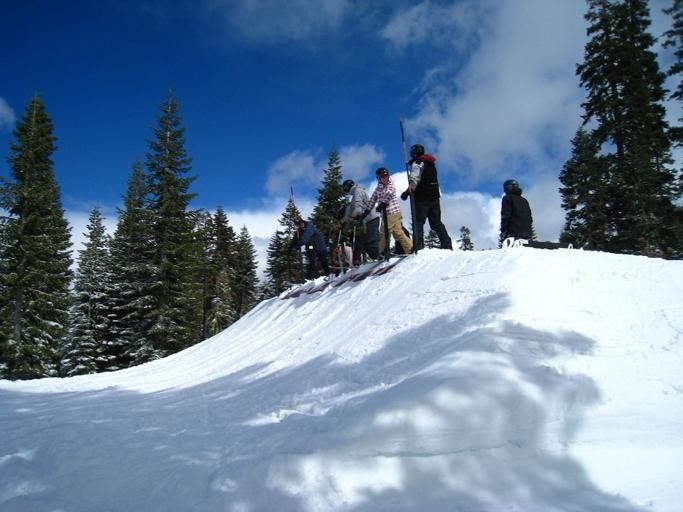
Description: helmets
xmin=343 ymin=180 xmax=354 ymax=192
xmin=411 ymin=144 xmax=424 ymax=157
xmin=505 ymin=180 xmax=519 ymax=193
xmin=377 ymin=168 xmax=389 ymax=185
xmin=336 ymin=205 xmax=345 ymax=214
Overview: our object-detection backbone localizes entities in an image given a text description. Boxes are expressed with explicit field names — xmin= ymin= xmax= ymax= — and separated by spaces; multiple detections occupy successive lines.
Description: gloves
xmin=401 ymin=191 xmax=409 ymax=200
xmin=376 ymin=202 xmax=386 ymax=212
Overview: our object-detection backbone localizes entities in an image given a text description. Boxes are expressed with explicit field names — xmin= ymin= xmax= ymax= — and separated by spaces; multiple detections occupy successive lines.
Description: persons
xmin=498 ymin=179 xmax=536 ymax=250
xmin=285 ymin=144 xmax=452 ymax=281
